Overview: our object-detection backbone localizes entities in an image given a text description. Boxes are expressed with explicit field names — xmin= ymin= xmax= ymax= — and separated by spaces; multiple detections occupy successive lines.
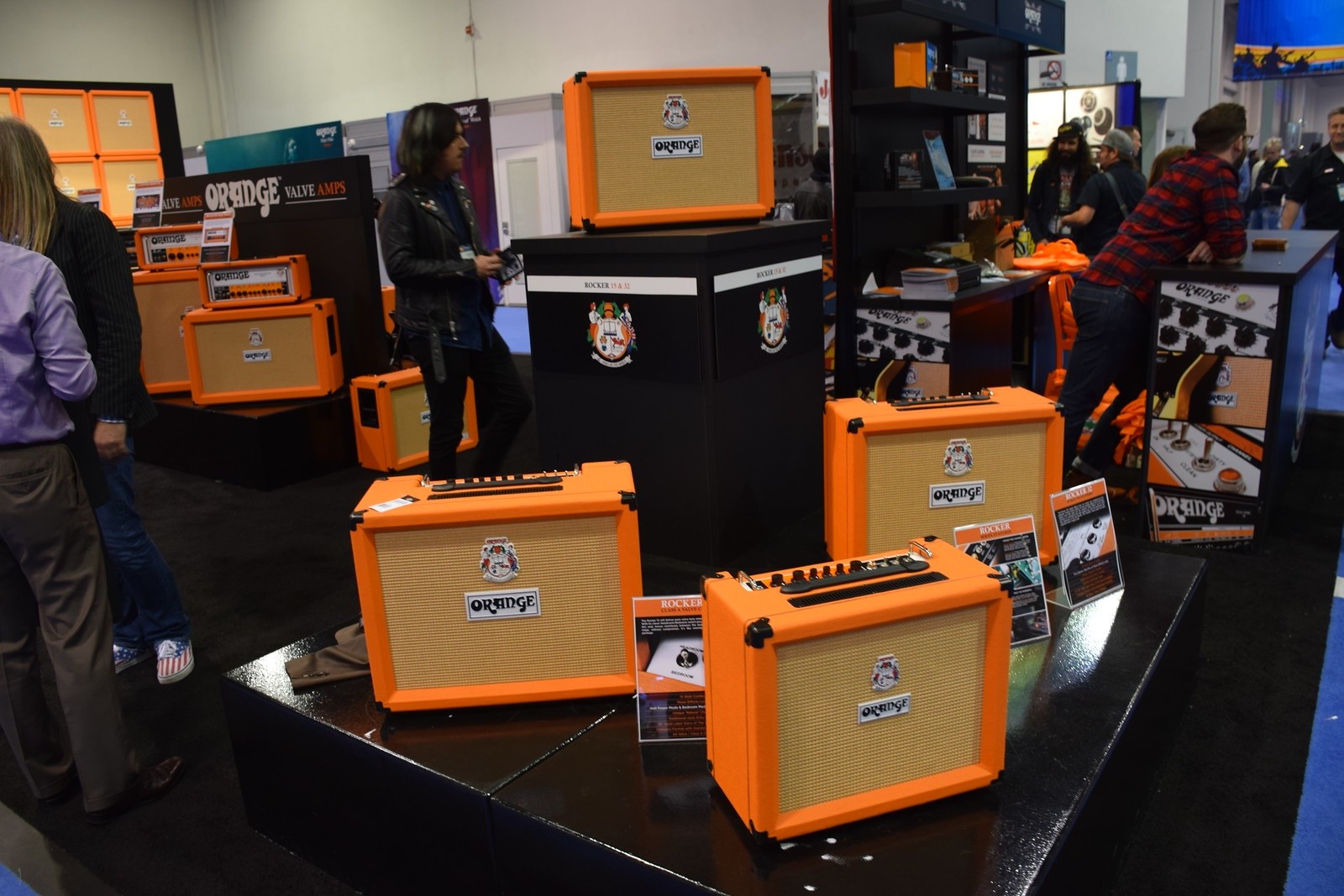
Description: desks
xmin=1138 ymin=228 xmax=1338 ymax=549
xmin=852 ymin=267 xmax=1059 ymax=407
xmin=508 ymin=218 xmax=827 ymax=571
xmin=218 ymin=532 xmax=1207 ymax=896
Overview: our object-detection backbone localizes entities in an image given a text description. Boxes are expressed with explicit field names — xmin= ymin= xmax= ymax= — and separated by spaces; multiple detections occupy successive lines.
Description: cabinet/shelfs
xmin=830 ymin=0 xmax=1066 ymax=275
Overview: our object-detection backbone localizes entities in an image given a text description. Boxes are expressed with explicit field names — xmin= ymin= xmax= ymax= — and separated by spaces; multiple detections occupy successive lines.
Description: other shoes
xmin=1065 ymin=471 xmax=1091 ymax=490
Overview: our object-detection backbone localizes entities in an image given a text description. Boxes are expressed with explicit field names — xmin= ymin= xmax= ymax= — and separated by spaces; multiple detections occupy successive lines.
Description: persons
xmin=376 ymin=103 xmax=531 ymax=479
xmin=1056 ymin=103 xmax=1247 ymax=485
xmin=1056 ymin=128 xmax=1149 ymax=254
xmin=0 ymin=115 xmax=200 ymax=814
xmin=1280 ymin=106 xmax=1344 ymax=360
xmin=1025 ymin=122 xmax=1322 ymax=254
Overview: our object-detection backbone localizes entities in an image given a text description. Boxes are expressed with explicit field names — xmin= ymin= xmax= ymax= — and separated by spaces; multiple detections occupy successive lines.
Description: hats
xmin=1093 ymin=129 xmax=1134 ymax=155
xmin=1052 ymin=122 xmax=1084 ymax=141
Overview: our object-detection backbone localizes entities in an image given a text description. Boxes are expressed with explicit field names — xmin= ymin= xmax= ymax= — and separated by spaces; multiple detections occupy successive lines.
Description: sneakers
xmin=114 ymin=644 xmax=152 ymax=676
xmin=155 ymin=640 xmax=195 ymax=684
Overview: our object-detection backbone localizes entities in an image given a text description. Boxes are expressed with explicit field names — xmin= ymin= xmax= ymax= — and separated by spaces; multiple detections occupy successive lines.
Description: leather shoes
xmin=92 ymin=756 xmax=184 ymax=822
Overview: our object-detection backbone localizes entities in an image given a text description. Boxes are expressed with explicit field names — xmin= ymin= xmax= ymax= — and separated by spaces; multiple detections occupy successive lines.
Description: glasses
xmin=1243 ymin=133 xmax=1253 ymax=145
